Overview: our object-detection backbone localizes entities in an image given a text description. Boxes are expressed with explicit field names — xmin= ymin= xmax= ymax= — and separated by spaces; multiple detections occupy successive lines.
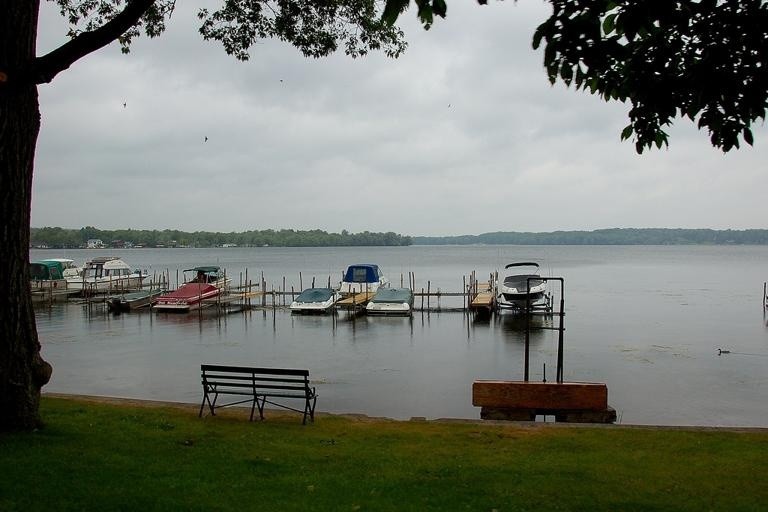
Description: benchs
xmin=198 ymin=363 xmax=320 ymax=427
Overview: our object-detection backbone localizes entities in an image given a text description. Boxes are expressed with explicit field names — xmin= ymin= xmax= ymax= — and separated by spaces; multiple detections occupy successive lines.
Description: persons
xmin=197 ymin=272 xmax=204 ymax=283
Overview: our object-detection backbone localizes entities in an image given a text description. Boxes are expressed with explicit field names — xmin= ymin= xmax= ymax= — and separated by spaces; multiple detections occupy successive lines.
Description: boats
xmin=30 ymin=257 xmax=149 ymax=302
xmin=366 ymin=288 xmax=414 ymax=317
xmin=178 ymin=266 xmax=232 ymax=290
xmin=106 ymin=284 xmax=224 ymax=312
xmin=498 ymin=262 xmax=549 ymax=316
xmin=337 ymin=264 xmax=386 ymax=298
xmin=289 ymin=288 xmax=342 ymax=315
